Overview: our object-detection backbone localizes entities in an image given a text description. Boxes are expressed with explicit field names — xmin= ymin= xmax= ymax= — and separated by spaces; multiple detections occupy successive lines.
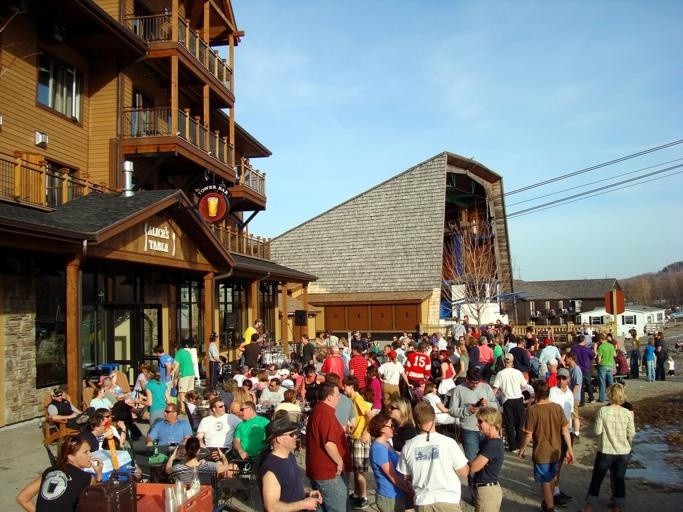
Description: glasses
xmin=379 ymin=424 xmax=393 ymax=428
xmin=104 ymin=415 xmax=110 ymax=418
xmin=391 ymin=405 xmax=398 ymax=410
xmin=71 ymin=436 xmax=81 ymax=445
xmin=283 ymin=432 xmax=296 ymax=437
xmin=269 ymin=384 xmax=275 ymax=387
xmin=54 ymin=394 xmax=62 ymax=398
xmin=165 ymin=410 xmax=177 ymax=412
xmin=219 ymin=405 xmax=224 ymax=409
xmin=240 ymin=407 xmax=250 ymax=411
xmin=477 ymin=418 xmax=483 ymax=423
xmin=557 ymin=376 xmax=566 ymax=380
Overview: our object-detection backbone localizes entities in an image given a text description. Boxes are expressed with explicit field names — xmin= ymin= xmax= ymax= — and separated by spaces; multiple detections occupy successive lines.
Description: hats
xmin=262 ymin=416 xmax=300 ymax=443
xmin=504 ymin=353 xmax=514 ymax=362
xmin=543 ymin=339 xmax=552 ymax=344
xmin=50 ymin=388 xmax=63 ymax=395
xmin=279 ymin=369 xmax=289 ymax=375
xmin=545 ymin=359 xmax=558 ymax=367
xmin=557 ymin=368 xmax=569 ymax=377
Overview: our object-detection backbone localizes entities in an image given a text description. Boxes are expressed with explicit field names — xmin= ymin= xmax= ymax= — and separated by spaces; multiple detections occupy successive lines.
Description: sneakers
xmin=573 ymin=435 xmax=580 ymax=444
xmin=541 ymin=491 xmax=622 ymax=511
xmin=352 ymin=498 xmax=369 ymax=509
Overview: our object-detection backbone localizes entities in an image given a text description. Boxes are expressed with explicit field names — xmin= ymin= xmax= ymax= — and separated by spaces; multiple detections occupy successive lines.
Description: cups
xmin=207 ymin=198 xmax=219 ymax=216
xmin=162 ymin=480 xmax=186 ymax=511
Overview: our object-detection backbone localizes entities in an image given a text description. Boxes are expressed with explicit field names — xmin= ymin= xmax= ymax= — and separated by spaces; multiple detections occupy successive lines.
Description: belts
xmin=477 ymin=481 xmax=497 ymax=486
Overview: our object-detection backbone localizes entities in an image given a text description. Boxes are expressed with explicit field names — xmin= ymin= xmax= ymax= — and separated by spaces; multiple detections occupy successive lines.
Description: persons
xmin=470 ymin=408 xmax=505 ymax=512
xmin=547 ymin=368 xmax=580 ymax=507
xmin=258 ymin=417 xmax=323 ymax=512
xmin=306 ymin=381 xmax=355 ymax=512
xmin=15 ymin=435 xmax=104 ymax=512
xmin=48 ymin=316 xmax=675 ymax=479
xmin=582 ymin=383 xmax=636 ymax=512
xmin=396 ymin=401 xmax=471 ymax=512
xmin=165 ymin=438 xmax=229 ymax=508
xmin=342 ymin=374 xmax=372 ymax=507
xmin=370 ymin=414 xmax=416 ymax=511
xmin=518 ymin=380 xmax=575 ymax=512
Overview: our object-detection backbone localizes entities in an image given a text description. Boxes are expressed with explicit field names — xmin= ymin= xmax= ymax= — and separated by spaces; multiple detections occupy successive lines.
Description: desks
xmin=137 ymin=482 xmax=214 ymax=512
xmin=134 ymin=444 xmax=230 ymax=481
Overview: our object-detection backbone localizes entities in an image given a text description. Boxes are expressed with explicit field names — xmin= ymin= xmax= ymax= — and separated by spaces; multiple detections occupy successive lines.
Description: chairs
xmin=289 ymin=411 xmax=303 ymax=452
xmin=169 ymin=470 xmax=218 ymax=509
xmin=230 ymin=403 xmax=274 ymax=501
xmin=82 ymin=387 xmax=96 ymax=413
xmin=109 ymin=370 xmax=131 ymax=393
xmin=42 ymin=391 xmax=80 ymax=447
xmin=182 ymin=401 xmax=201 ymax=436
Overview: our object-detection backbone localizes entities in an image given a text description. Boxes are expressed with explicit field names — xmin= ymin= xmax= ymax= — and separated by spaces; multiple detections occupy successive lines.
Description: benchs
xmin=592 ymin=374 xmax=627 ymax=393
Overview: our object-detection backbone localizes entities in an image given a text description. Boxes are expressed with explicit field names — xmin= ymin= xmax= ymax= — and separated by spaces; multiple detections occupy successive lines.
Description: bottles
xmin=135 ymin=390 xmax=139 ymax=408
xmin=153 ymin=439 xmax=160 ymax=457
xmin=190 ymin=465 xmax=200 ymax=495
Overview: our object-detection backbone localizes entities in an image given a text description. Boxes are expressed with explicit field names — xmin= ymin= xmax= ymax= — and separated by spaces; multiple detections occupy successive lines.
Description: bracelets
xmin=95 ymin=479 xmax=105 ymax=483
